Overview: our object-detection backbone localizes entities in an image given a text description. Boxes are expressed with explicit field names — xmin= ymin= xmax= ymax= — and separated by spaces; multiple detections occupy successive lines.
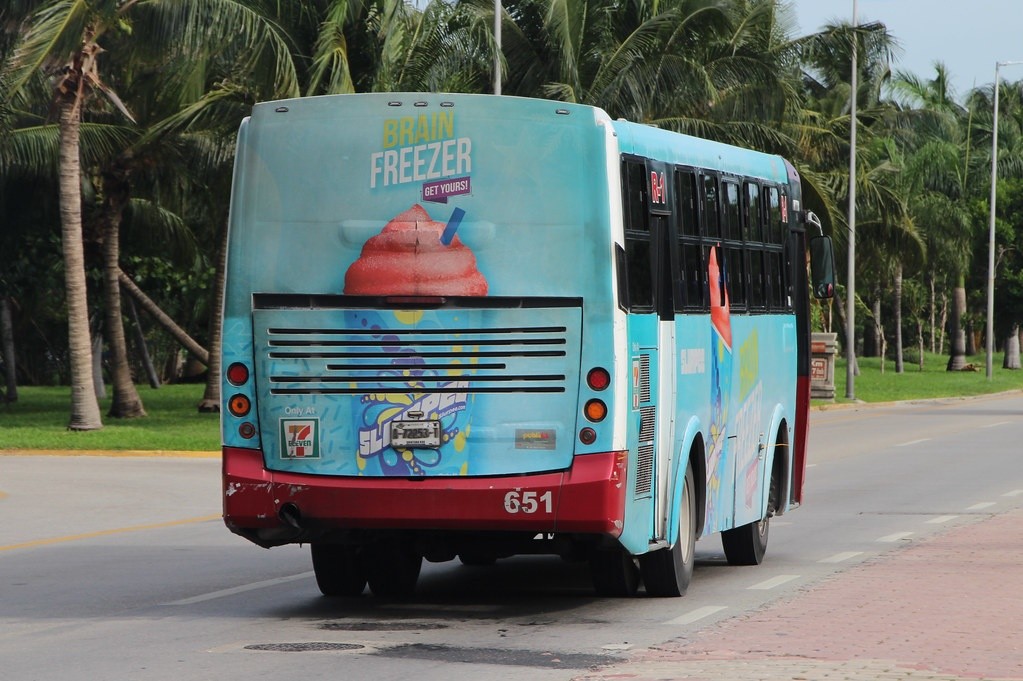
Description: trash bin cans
xmin=810 ymin=331 xmax=839 ymax=398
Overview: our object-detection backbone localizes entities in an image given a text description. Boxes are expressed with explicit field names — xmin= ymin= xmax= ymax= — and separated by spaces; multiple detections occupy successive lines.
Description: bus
xmin=220 ymin=92 xmax=835 ymax=596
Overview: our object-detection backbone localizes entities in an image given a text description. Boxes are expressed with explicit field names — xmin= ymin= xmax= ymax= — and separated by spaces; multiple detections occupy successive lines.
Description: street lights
xmin=985 ymin=61 xmax=1023 ymax=381
